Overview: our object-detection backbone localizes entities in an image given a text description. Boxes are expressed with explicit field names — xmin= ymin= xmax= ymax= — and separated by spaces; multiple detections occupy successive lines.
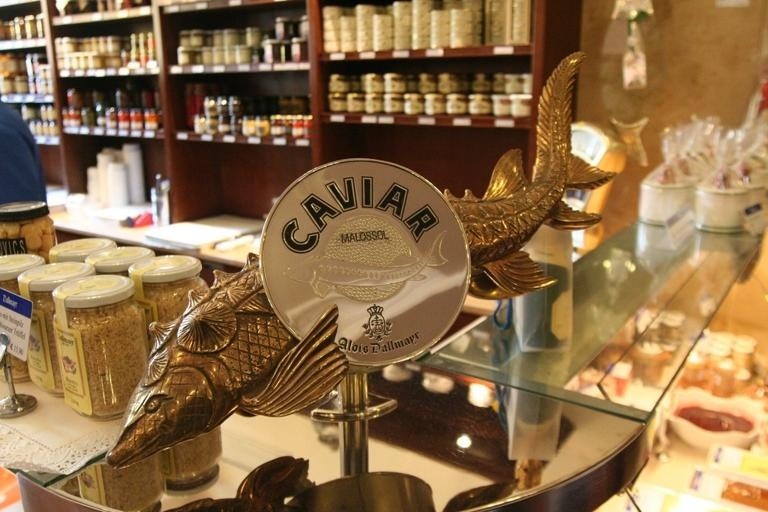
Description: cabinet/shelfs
xmin=1 ymin=0 xmax=584 ymax=487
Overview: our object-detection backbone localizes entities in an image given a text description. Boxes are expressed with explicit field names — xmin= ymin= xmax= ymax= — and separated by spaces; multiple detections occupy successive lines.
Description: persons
xmin=0 ymin=93 xmax=48 ymax=203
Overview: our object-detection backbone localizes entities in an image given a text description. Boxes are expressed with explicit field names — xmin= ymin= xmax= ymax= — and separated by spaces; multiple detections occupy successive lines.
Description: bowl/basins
xmin=663 ymin=396 xmax=762 ymax=454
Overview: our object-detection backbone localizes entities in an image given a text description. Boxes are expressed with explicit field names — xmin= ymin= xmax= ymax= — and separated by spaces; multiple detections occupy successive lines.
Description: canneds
xmin=52 ymin=275 xmax=147 ymax=421
xmin=321 ymin=0 xmax=531 ymax=52
xmin=0 ymin=52 xmax=27 ymax=93
xmin=72 ymin=428 xmax=223 ymax=510
xmin=328 ymin=70 xmax=533 ymax=115
xmin=1 ymin=254 xmax=45 ymax=383
xmin=20 ymin=104 xmax=58 ymax=137
xmin=577 ymin=307 xmax=756 ymax=399
xmin=62 ymin=86 xmax=163 ymax=130
xmin=192 ymin=96 xmax=314 ymax=137
xmin=0 ymin=201 xmax=57 ymax=264
xmin=178 ymin=14 xmax=309 ymax=65
xmin=127 ymin=255 xmax=208 ymax=323
xmin=54 ymin=31 xmax=130 ymax=70
xmin=17 ymin=262 xmax=97 ymax=397
xmin=0 ymin=13 xmax=46 ymax=41
xmin=48 ymin=239 xmax=155 ymax=276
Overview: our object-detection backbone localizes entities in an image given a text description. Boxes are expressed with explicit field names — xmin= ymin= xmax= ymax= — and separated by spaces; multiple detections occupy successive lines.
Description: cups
xmin=150 ymin=186 xmax=169 ymax=227
xmin=86 ymin=144 xmax=147 ymax=212
xmin=637 ymin=175 xmax=766 ymax=234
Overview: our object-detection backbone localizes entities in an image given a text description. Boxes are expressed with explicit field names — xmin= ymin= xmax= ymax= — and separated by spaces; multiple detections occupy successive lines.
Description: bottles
xmin=682 ymin=329 xmax=759 ymax=398
xmin=634 ymin=308 xmax=686 ymax=366
